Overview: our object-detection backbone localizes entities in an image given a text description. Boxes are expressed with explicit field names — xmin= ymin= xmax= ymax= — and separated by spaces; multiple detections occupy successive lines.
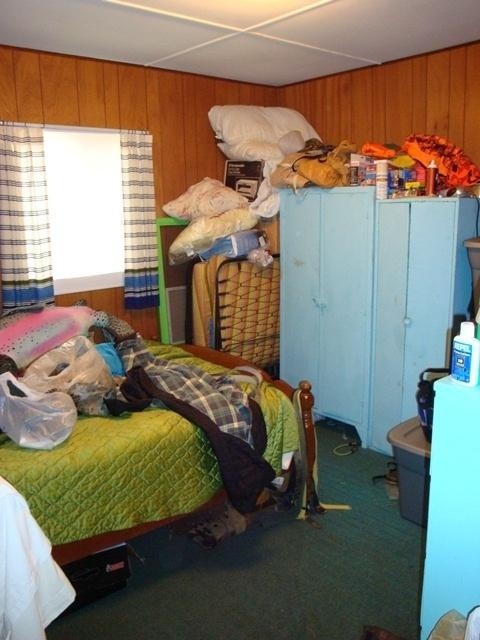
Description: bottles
xmin=425 ymin=160 xmax=437 ymax=197
xmin=375 ymin=163 xmax=388 ymax=200
xmin=452 ymin=321 xmax=479 ymax=383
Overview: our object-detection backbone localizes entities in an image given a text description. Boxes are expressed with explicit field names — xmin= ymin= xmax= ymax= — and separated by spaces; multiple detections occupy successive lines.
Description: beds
xmin=1 ymin=345 xmax=316 ymax=567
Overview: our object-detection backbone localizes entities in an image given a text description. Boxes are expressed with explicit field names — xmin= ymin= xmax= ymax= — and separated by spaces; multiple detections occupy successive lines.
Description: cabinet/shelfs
xmin=280 ymin=187 xmax=480 ymax=460
xmin=419 ymin=376 xmax=480 ymax=639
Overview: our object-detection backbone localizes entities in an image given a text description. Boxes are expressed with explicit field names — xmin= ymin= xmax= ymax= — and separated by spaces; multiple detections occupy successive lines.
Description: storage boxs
xmin=386 ymin=417 xmax=430 ymax=528
